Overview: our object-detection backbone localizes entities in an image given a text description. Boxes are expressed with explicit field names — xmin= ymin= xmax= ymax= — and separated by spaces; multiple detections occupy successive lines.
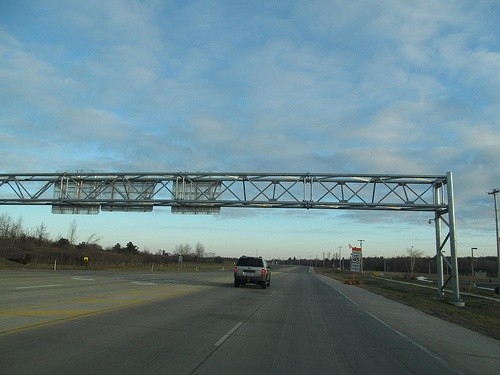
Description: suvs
xmin=233 ymin=255 xmax=272 ymax=288
